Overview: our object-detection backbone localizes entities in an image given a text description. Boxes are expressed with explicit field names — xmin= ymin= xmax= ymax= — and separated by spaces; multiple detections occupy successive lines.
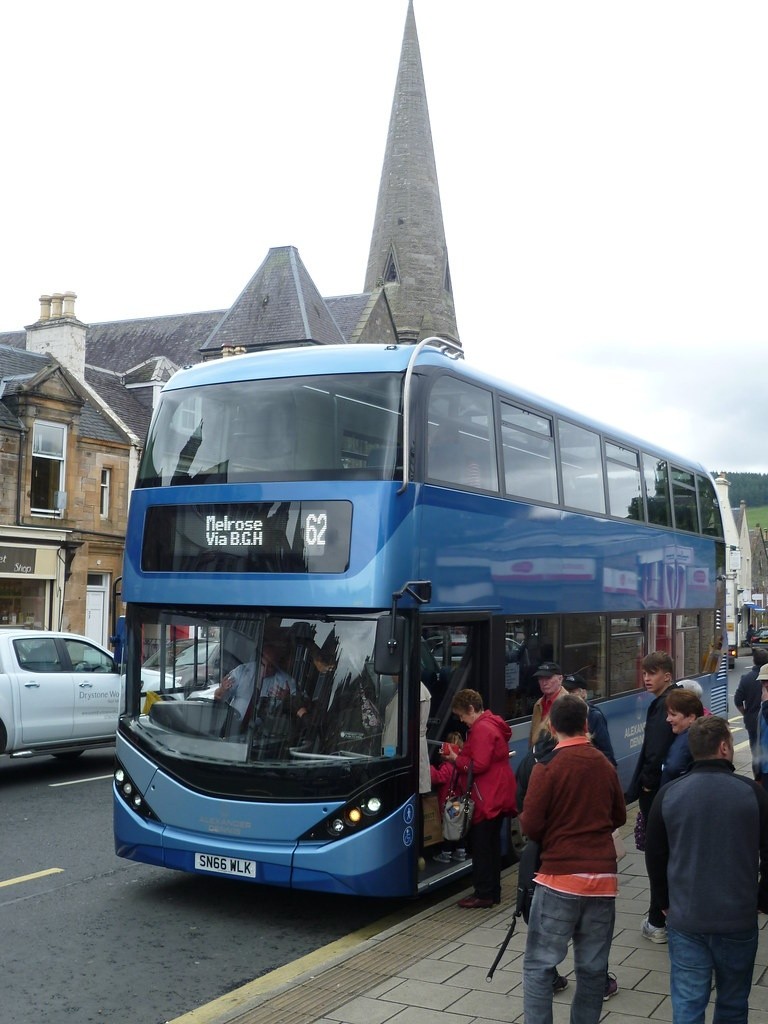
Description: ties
xmin=240 ymin=675 xmax=264 ymax=733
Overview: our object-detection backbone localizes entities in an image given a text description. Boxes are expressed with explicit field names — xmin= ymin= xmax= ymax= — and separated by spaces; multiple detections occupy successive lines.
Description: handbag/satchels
xmin=441 ymin=756 xmax=476 ymax=841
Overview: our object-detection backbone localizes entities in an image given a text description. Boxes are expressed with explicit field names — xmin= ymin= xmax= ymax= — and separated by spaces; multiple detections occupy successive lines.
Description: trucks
xmin=726 ymin=572 xmax=741 ymax=668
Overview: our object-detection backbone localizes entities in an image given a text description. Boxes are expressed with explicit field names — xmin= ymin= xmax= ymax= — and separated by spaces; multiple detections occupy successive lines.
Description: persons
xmin=528 ymin=662 xmax=569 ymax=752
xmin=214 ymin=646 xmax=768 ymax=1024
xmin=441 ymin=688 xmax=519 ymax=909
xmin=520 ymin=694 xmax=627 ymax=1024
xmin=626 ymin=650 xmax=684 ymax=944
xmin=645 ymin=715 xmax=768 ymax=1024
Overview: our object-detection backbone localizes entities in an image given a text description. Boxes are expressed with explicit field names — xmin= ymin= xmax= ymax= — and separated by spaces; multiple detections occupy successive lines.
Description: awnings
xmin=744 ymin=604 xmax=768 ymax=612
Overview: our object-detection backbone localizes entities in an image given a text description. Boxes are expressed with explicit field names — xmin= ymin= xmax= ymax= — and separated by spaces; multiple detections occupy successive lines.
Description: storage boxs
xmin=422 ymin=795 xmax=443 ymax=847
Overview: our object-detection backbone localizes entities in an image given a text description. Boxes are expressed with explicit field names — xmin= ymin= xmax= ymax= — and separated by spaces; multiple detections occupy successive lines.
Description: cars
xmin=148 ymin=638 xmax=220 ymax=689
xmin=431 ymin=637 xmax=523 ymax=672
xmin=749 ymin=626 xmax=768 ymax=654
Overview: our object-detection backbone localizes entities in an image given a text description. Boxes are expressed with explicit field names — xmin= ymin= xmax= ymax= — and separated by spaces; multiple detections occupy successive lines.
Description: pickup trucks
xmin=0 ymin=626 xmax=183 ymax=761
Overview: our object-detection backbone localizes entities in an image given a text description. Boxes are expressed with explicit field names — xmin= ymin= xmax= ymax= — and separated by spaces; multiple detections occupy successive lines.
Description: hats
xmin=756 ymin=663 xmax=768 ymax=680
xmin=531 ymin=662 xmax=561 ymax=679
xmin=562 ymin=674 xmax=587 ymax=692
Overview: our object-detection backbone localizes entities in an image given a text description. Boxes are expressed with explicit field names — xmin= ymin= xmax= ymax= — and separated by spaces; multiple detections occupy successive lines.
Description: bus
xmin=111 ymin=329 xmax=729 ymax=899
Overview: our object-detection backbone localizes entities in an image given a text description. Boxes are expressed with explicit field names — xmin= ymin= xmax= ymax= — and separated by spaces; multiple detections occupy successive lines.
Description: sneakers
xmin=602 ymin=973 xmax=618 ymax=1000
xmin=452 ymin=850 xmax=467 ymax=862
xmin=433 ymin=852 xmax=452 ymax=862
xmin=640 ymin=917 xmax=669 ymax=944
xmin=552 ymin=972 xmax=569 ymax=997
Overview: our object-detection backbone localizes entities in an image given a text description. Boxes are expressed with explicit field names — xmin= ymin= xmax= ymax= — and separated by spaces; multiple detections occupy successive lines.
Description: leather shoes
xmin=458 ymin=890 xmax=494 ymax=909
